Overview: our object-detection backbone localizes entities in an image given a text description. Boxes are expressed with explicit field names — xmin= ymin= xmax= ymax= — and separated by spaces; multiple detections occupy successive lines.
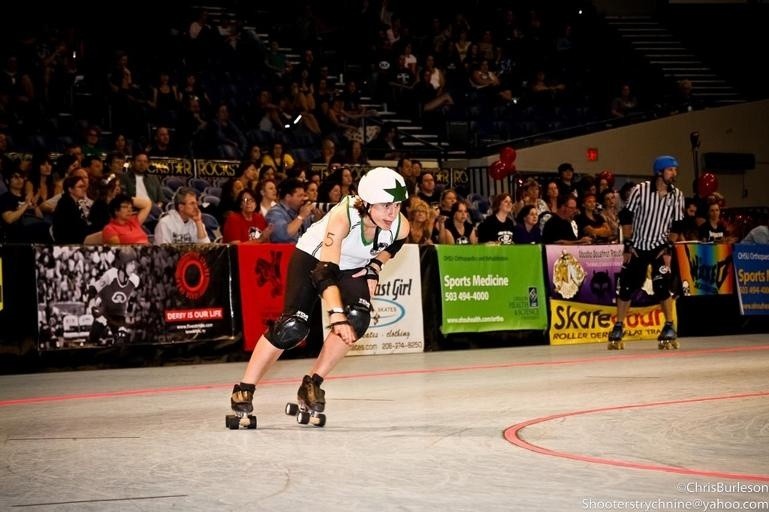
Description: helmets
xmin=653 ymin=156 xmax=679 ymax=173
xmin=357 ymin=167 xmax=409 ymax=204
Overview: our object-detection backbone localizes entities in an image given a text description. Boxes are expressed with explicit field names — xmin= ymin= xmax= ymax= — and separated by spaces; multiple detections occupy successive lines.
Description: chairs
xmin=142 ymin=215 xmax=158 ymax=234
xmin=162 ymin=186 xmax=174 ymax=201
xmin=188 ymin=178 xmax=211 ymax=188
xmin=468 ymin=192 xmax=492 ymax=215
xmin=165 ymin=202 xmax=175 ymax=211
xmin=163 ymin=177 xmax=184 ymax=192
xmin=204 ymin=186 xmax=222 ymax=196
xmin=201 ymin=194 xmax=220 ymax=208
xmin=202 ymin=213 xmax=220 ymax=242
xmin=146 ymin=205 xmax=163 ymax=219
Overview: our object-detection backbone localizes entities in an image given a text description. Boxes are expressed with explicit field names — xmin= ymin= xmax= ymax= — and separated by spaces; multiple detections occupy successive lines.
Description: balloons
xmin=698 ymin=171 xmax=719 ymax=200
xmin=499 ymin=147 xmax=517 ymax=165
xmin=708 ymin=192 xmax=725 ymax=213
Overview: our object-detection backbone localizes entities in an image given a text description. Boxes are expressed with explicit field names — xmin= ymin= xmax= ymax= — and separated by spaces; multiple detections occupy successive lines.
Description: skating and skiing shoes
xmin=608 ymin=325 xmax=624 ymax=350
xmin=226 ymin=384 xmax=256 ymax=429
xmin=658 ymin=325 xmax=680 ymax=349
xmin=286 ymin=374 xmax=325 ymax=426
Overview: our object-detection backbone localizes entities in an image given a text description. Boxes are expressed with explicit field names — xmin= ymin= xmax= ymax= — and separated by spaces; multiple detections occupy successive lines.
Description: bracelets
xmin=371 ymin=259 xmax=384 ymax=271
xmin=328 ymin=307 xmax=344 ymax=316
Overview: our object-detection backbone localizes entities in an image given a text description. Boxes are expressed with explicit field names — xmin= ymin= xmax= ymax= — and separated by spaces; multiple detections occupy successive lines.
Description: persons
xmin=388 ymin=2 xmax=714 ymax=160
xmin=682 ymin=197 xmax=768 ymax=244
xmin=229 ymin=163 xmax=412 ymax=414
xmin=1 ymin=1 xmax=404 ymax=166
xmin=1 ymin=155 xmax=633 ymax=245
xmin=607 ymin=155 xmax=685 ymax=341
